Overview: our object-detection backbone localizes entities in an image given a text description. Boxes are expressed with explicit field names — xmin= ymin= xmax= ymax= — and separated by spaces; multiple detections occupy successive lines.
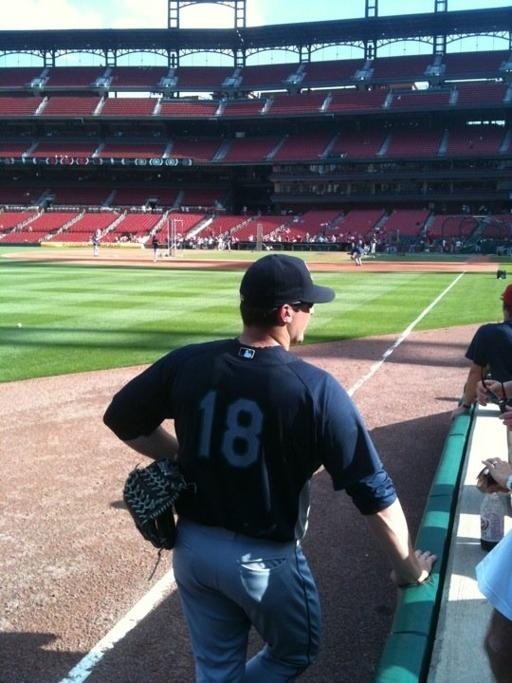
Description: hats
xmin=240 ymin=253 xmax=334 ymax=308
xmin=500 ymin=284 xmax=512 ymax=305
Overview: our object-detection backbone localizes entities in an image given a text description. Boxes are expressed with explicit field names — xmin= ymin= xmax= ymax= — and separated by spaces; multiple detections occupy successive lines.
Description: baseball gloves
xmin=123 ymin=452 xmax=197 ymax=550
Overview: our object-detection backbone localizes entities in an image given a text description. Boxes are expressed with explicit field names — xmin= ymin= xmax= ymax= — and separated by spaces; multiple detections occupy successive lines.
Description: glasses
xmin=479 ymin=362 xmax=507 ymax=404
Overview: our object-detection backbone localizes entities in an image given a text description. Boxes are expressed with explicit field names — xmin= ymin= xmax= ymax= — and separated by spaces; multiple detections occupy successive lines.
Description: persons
xmin=102 ymin=255 xmax=437 ymax=683
xmin=450 ymin=285 xmax=511 ymax=421
xmin=475 ymin=529 xmax=511 ymax=683
xmin=476 ymin=379 xmax=512 ymax=432
xmin=477 ymin=456 xmax=512 ymax=493
xmin=1 ymin=203 xmax=511 ymax=267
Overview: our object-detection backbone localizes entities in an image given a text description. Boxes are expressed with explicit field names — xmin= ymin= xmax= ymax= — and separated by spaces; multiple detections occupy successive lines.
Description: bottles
xmin=479 ymin=490 xmax=506 ymax=553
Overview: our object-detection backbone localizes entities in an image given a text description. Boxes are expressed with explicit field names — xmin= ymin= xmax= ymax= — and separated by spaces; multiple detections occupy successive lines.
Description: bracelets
xmin=457 ymin=400 xmax=469 ymax=409
xmin=506 ymin=474 xmax=512 ymax=490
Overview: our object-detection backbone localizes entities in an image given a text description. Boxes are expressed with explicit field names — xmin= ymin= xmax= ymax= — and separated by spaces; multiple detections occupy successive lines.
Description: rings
xmin=493 ymin=460 xmax=497 ymax=465
